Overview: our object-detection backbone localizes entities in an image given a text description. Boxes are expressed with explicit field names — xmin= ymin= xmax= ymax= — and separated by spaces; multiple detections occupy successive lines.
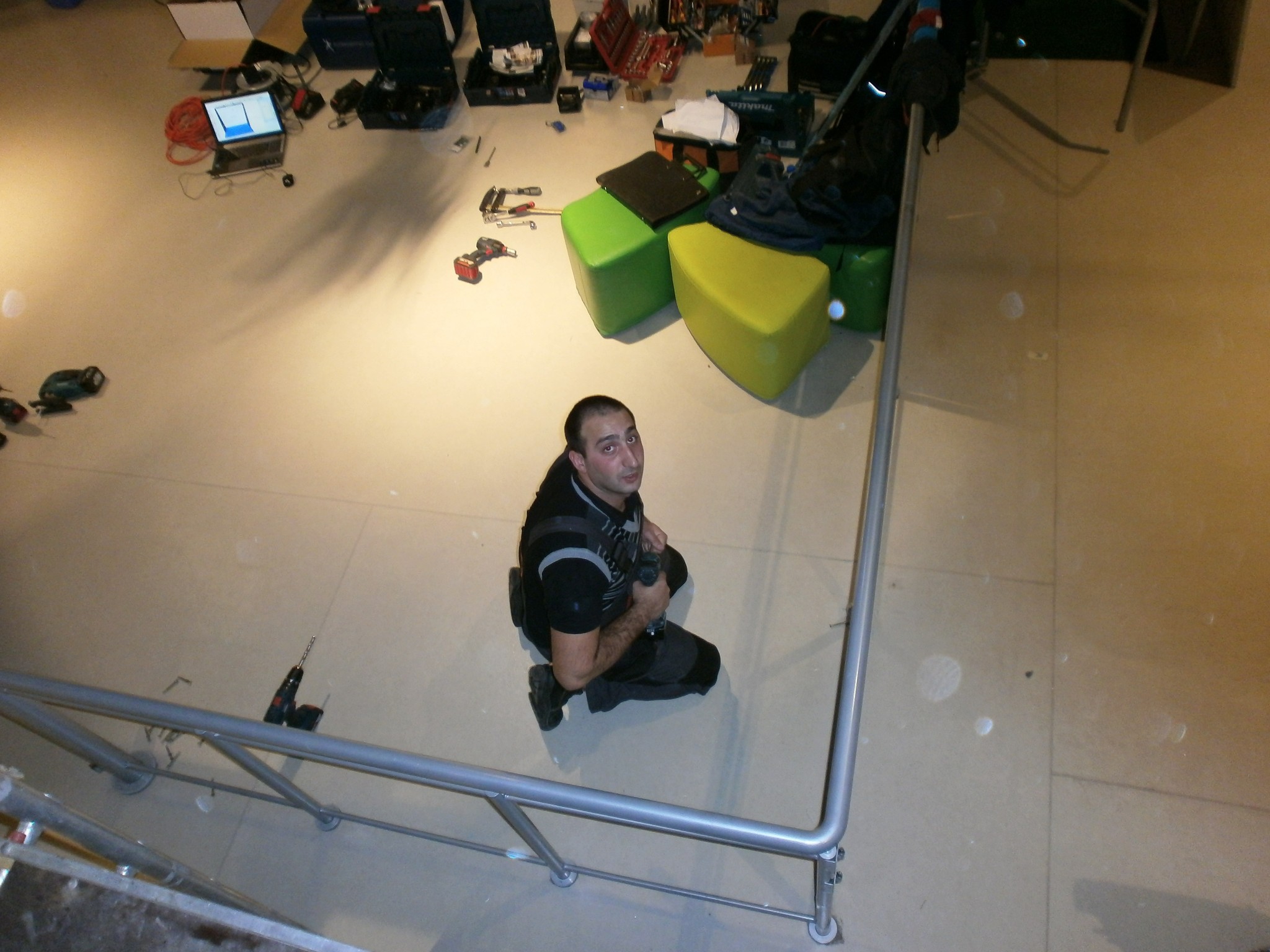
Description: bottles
xmin=780 ymin=165 xmax=795 ymax=185
xmin=639 ymin=567 xmax=667 ymax=634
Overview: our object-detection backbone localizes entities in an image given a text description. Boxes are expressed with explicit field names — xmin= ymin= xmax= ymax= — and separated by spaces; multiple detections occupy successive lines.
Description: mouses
xmin=282 ymin=174 xmax=294 ymax=187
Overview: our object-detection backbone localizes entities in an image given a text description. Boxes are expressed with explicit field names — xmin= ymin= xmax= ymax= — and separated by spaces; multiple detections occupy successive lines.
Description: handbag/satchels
xmin=596 ymin=150 xmax=710 ymax=229
xmin=709 ymin=145 xmax=824 ymax=252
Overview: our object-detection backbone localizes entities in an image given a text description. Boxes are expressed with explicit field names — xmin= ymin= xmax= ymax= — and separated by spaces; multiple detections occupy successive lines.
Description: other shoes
xmin=528 ymin=663 xmax=570 ymax=732
xmin=508 ymin=566 xmax=525 ymax=627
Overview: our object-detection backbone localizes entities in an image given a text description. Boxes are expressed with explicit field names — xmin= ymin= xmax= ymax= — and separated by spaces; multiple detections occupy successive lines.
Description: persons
xmin=509 ymin=395 xmax=721 ymax=729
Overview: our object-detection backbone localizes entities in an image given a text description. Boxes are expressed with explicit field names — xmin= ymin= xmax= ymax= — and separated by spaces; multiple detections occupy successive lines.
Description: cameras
xmin=638 ymin=553 xmax=668 ymax=638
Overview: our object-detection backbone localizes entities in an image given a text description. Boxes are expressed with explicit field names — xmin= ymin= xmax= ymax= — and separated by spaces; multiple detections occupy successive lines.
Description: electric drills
xmin=453 ymin=237 xmax=516 ymax=282
xmin=262 ymin=634 xmax=323 ymax=731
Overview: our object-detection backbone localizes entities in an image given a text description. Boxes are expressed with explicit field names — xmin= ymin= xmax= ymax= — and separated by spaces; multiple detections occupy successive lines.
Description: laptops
xmin=201 ymin=89 xmax=286 ymax=178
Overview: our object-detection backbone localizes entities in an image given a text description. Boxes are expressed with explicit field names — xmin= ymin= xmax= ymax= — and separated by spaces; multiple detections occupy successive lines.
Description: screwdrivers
xmin=494 ymin=201 xmax=535 ymax=216
xmin=498 ymin=187 xmax=542 ymax=195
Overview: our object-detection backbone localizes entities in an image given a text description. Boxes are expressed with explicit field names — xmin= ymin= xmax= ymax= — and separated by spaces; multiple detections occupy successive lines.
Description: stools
xmin=560 ymin=167 xmax=721 ymax=338
xmin=669 ymin=222 xmax=833 ymax=403
xmin=816 ymin=236 xmax=893 ymax=333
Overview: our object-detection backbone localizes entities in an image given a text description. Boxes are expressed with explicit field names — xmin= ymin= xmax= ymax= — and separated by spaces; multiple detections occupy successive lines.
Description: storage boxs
xmin=303 ymin=0 xmax=375 ymax=70
xmin=355 ymin=3 xmax=460 ymax=131
xmin=168 ymin=0 xmax=311 ymax=76
xmin=710 ymin=88 xmax=814 ymax=160
xmin=464 ymin=0 xmax=561 ymax=108
xmin=563 ymin=10 xmax=610 ymax=77
xmin=588 ymin=0 xmax=687 ymax=84
xmin=653 ymin=110 xmax=742 ymax=175
